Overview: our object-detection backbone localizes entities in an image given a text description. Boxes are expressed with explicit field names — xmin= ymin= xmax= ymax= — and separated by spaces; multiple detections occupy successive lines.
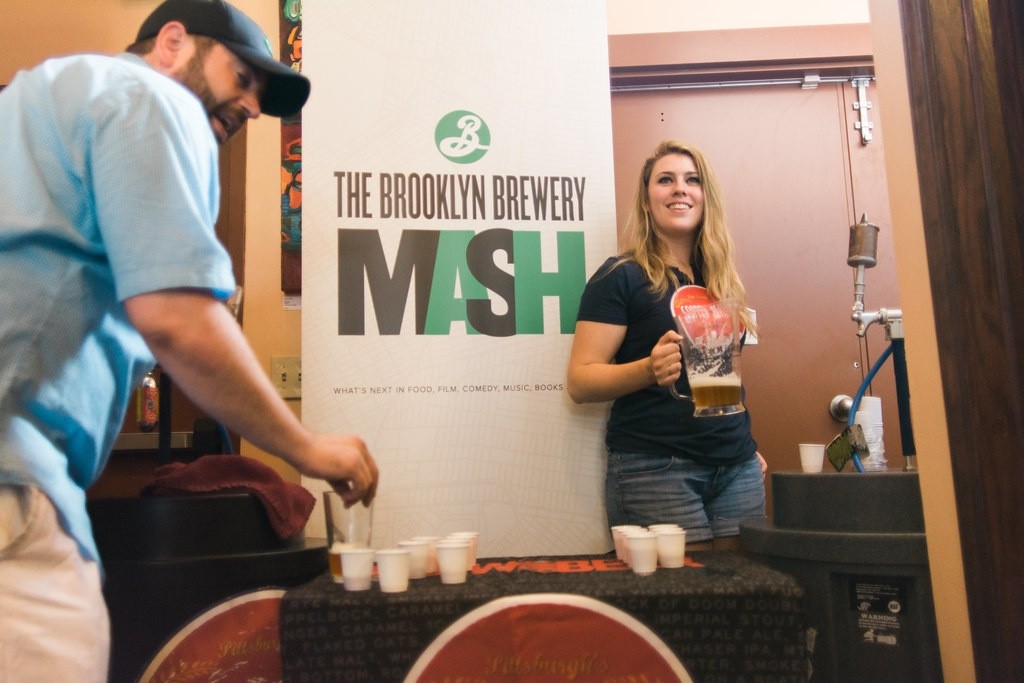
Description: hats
xmin=135 ymin=0 xmax=311 ymax=118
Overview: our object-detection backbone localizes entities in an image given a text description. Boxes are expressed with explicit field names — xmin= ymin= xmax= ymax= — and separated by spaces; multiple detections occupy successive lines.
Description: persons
xmin=0 ymin=0 xmax=379 ymax=683
xmin=567 ymin=142 xmax=769 ymax=552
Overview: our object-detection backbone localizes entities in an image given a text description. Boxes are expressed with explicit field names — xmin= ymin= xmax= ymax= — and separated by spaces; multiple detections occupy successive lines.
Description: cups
xmin=799 ymin=444 xmax=825 ymax=473
xmin=855 ymin=397 xmax=888 ymax=472
xmin=611 ymin=524 xmax=687 ymax=576
xmin=341 ymin=532 xmax=478 ymax=592
xmin=669 ymin=301 xmax=745 ymax=417
xmin=324 ymin=491 xmax=372 ymax=583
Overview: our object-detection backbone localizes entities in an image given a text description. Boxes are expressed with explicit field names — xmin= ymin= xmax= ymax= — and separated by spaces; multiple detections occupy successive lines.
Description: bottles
xmin=137 ymin=372 xmax=159 ymax=432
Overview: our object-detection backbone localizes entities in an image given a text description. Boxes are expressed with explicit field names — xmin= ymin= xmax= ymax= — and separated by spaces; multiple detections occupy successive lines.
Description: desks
xmin=280 ymin=549 xmax=809 ymax=683
xmin=737 ymin=469 xmax=948 ymax=683
xmin=85 ymin=490 xmax=328 ymax=683
xmin=84 ymin=431 xmax=195 ymax=492
